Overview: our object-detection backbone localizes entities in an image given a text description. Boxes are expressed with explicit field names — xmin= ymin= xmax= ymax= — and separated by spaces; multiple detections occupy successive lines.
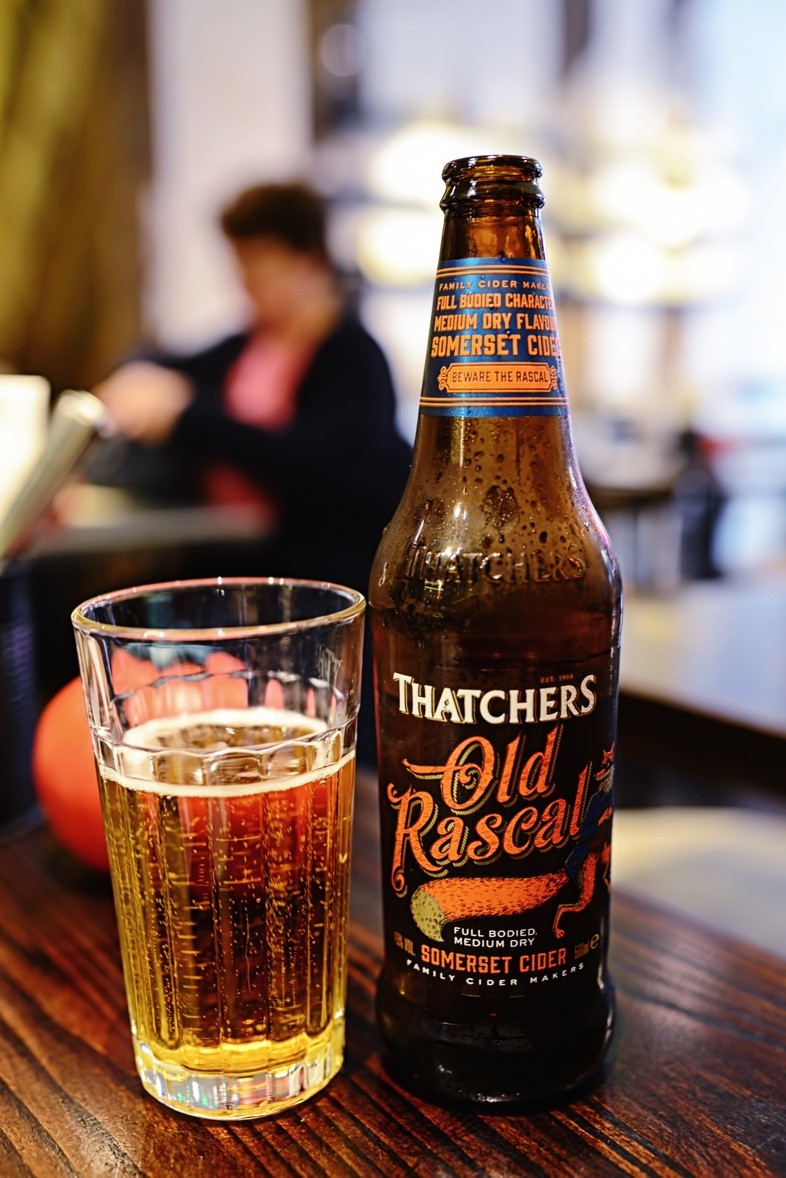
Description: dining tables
xmin=2 ymin=762 xmax=784 ymax=1178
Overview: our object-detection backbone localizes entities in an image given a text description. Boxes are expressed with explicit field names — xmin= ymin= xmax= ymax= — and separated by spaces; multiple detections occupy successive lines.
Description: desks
xmin=617 ymin=581 xmax=785 ymax=804
xmin=0 ymin=457 xmax=268 ymax=792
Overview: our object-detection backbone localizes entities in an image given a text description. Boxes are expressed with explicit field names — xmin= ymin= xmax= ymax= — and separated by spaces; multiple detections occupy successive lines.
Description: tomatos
xmin=33 ymin=637 xmax=328 ymax=880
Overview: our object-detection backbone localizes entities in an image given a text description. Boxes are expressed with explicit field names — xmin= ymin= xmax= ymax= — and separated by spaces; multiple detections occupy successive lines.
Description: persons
xmin=98 ymin=184 xmax=413 ymax=585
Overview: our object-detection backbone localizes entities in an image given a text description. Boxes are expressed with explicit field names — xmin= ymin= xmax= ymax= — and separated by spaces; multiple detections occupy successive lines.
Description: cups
xmin=368 ymin=150 xmax=619 ymax=1109
xmin=71 ymin=572 xmax=372 ymax=1124
